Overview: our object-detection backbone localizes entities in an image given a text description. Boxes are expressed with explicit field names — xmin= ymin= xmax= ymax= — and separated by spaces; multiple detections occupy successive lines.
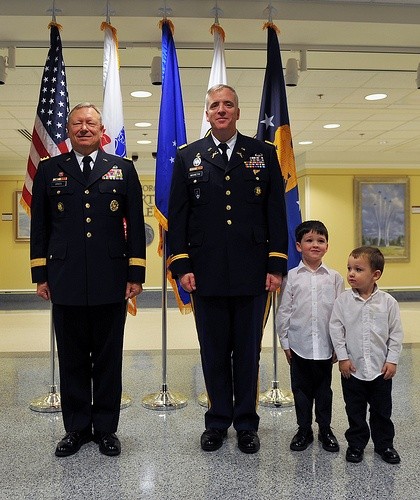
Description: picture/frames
xmin=351 ymin=173 xmax=410 ymax=264
xmin=12 ymin=190 xmax=31 ymax=243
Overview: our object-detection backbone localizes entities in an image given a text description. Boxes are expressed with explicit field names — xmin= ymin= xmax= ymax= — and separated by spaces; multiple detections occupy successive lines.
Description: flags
xmin=255 ymin=24 xmax=308 ymax=337
xmin=19 ymin=15 xmax=72 ymax=214
xmin=90 ymin=19 xmax=143 ymax=316
xmin=196 ymin=21 xmax=236 ymax=139
xmin=146 ymin=16 xmax=194 ymax=318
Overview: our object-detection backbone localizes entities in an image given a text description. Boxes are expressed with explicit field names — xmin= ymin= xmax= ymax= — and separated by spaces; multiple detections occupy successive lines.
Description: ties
xmin=81 ymin=156 xmax=94 ymax=179
xmin=217 ymin=142 xmax=230 ymax=166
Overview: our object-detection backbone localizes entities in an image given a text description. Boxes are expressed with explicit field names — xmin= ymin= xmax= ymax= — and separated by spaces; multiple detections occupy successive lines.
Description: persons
xmin=273 ymin=220 xmax=346 ymax=452
xmin=328 ymin=247 xmax=405 ymax=464
xmin=164 ymin=84 xmax=288 ymax=454
xmin=29 ymin=103 xmax=146 ymax=460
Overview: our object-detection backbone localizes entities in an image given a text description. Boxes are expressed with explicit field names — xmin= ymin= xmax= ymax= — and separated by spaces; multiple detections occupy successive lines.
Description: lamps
xmin=0 ymin=45 xmax=17 ymax=86
xmin=150 ymin=56 xmax=163 ymax=86
xmin=286 ymin=49 xmax=308 ymax=87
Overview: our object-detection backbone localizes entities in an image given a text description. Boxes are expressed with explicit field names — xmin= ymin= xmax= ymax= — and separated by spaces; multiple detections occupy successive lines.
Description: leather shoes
xmin=290 ymin=427 xmax=314 ymax=451
xmin=346 ymin=445 xmax=364 ymax=462
xmin=55 ymin=431 xmax=94 ymax=457
xmin=318 ymin=426 xmax=339 ymax=452
xmin=236 ymin=431 xmax=260 ymax=454
xmin=93 ymin=433 xmax=121 ymax=456
xmin=374 ymin=447 xmax=400 ymax=464
xmin=200 ymin=429 xmax=228 ymax=451
